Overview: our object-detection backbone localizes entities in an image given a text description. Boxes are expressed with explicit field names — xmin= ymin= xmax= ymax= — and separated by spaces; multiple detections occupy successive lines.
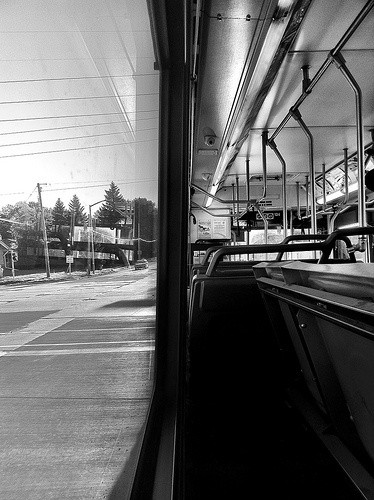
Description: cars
xmin=135 ymin=258 xmax=149 ymax=270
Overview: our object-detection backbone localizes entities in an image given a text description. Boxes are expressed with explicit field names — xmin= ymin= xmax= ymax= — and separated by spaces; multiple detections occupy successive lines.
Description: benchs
xmin=190 ymin=224 xmax=374 ymax=496
